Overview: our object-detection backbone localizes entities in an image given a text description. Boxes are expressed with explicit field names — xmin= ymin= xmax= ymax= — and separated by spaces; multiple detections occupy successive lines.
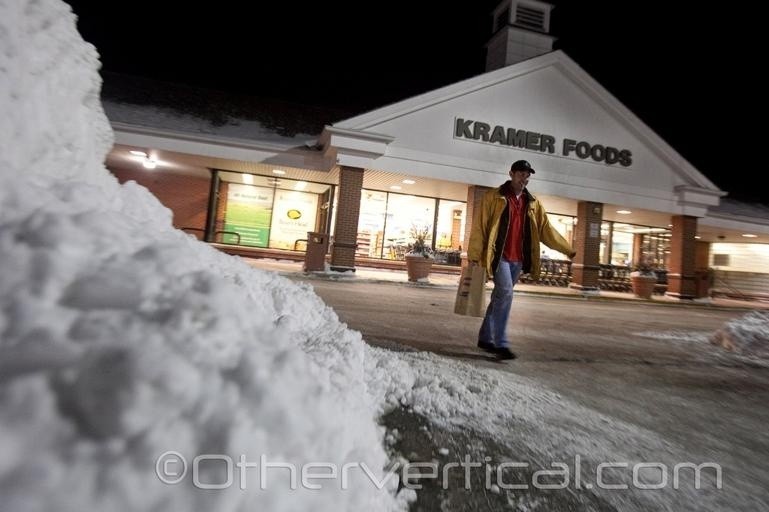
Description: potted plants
xmin=629 ymin=255 xmax=658 ymax=298
xmin=405 ymin=221 xmax=434 ymax=282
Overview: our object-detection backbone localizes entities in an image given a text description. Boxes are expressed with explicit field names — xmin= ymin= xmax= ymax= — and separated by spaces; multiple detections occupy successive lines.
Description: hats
xmin=511 ymin=161 xmax=535 ymax=173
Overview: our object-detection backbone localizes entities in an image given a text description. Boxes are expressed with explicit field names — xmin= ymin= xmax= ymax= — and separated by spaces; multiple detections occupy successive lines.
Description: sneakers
xmin=478 ymin=340 xmax=514 ymax=359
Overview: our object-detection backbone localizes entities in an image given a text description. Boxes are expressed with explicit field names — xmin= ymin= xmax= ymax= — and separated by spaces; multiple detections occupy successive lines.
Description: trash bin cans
xmin=303 ymin=231 xmax=330 ymax=272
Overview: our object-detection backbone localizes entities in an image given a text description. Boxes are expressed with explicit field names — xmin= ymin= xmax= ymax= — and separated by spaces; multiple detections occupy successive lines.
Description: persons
xmin=467 ymin=160 xmax=576 ymax=359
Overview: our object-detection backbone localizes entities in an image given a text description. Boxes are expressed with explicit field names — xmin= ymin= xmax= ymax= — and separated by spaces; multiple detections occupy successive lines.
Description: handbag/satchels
xmin=455 ymin=268 xmax=485 ymax=317
